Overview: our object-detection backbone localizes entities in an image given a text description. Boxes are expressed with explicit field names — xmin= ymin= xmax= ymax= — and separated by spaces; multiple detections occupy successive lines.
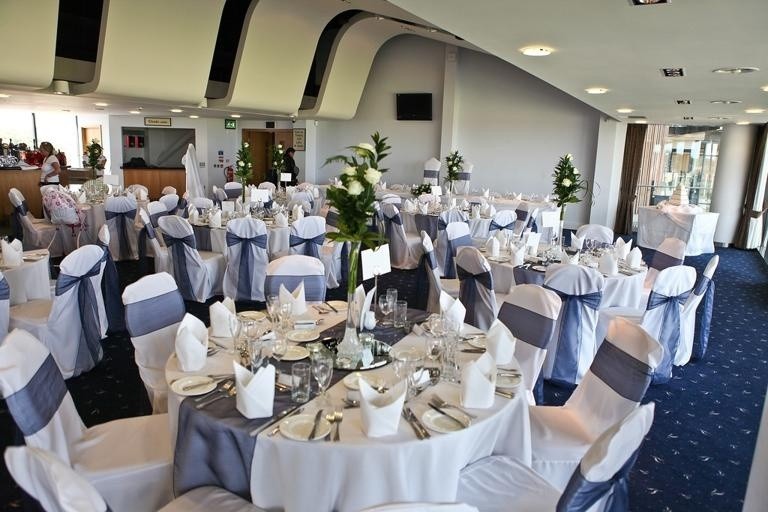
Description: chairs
xmin=0 ymin=158 xmax=720 ymax=512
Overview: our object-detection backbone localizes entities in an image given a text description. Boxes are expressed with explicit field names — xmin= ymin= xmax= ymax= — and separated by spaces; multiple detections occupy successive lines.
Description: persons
xmin=39 ymin=142 xmax=62 ymax=218
xmin=283 ymin=147 xmax=298 ymax=187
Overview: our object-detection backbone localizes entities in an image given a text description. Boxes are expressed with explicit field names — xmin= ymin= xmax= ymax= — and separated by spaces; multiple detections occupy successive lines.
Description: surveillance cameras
xmin=292 ymin=118 xmax=296 ymax=123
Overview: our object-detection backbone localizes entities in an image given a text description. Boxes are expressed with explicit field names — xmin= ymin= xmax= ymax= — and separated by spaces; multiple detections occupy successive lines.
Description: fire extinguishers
xmin=224 ymin=163 xmax=234 ymax=182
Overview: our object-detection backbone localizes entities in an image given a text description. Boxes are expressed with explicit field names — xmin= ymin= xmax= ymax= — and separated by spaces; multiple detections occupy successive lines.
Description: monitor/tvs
xmin=396 ymin=93 xmax=432 ymax=121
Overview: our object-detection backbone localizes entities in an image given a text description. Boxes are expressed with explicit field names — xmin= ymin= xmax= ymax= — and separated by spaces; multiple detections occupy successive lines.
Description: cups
xmin=388 ymin=289 xmax=400 ymax=313
xmin=393 ymin=301 xmax=408 ymax=326
xmin=289 ymin=363 xmax=309 ymax=403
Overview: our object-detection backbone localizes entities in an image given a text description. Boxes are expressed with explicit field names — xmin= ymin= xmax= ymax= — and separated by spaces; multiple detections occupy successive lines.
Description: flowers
xmin=234 ymin=141 xmax=255 ymax=203
xmin=317 ymin=131 xmax=395 ymax=294
xmin=272 ymin=144 xmax=285 ymax=171
xmin=82 ymin=138 xmax=104 ymax=165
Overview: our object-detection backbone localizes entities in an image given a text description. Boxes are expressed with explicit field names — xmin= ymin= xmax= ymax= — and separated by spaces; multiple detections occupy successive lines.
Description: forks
xmin=190 ymin=381 xmax=236 ymax=411
xmin=325 ymin=406 xmax=344 ymax=443
xmin=431 ymin=393 xmax=478 ymax=420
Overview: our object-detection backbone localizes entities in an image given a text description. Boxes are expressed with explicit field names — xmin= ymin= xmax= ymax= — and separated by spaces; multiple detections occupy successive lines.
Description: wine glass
xmin=391 ymin=314 xmax=461 ymax=399
xmin=199 ymin=204 xmax=290 ymax=226
xmin=504 ymin=226 xmax=617 ymax=273
xmin=376 ymin=293 xmax=393 ymax=326
xmin=310 ymin=352 xmax=334 ymax=404
xmin=227 ymin=292 xmax=292 ymax=371
xmin=68 ymin=186 xmax=127 ymax=205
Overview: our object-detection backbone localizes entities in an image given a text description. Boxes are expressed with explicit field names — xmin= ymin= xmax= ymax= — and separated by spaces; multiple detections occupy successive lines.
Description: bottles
xmin=0 ymin=138 xmax=3 ymax=156
xmin=8 ymin=138 xmax=14 ymax=154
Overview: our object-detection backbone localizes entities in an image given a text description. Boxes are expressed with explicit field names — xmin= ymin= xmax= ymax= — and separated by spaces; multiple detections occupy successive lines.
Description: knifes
xmin=306 ymin=409 xmax=323 ymax=441
xmin=427 ymin=402 xmax=467 ymax=429
xmin=248 ymin=405 xmax=304 ymax=438
xmin=400 ymin=406 xmax=430 ymax=441
xmin=322 ymin=300 xmax=338 ymax=313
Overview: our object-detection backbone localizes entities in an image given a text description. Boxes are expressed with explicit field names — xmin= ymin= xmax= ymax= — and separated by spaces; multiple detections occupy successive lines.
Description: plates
xmin=281 ymin=345 xmax=310 ymax=363
xmin=421 ymin=408 xmax=471 ymax=435
xmin=169 ymin=375 xmax=217 ymax=398
xmin=467 ymin=335 xmax=486 ymax=350
xmin=495 ymin=366 xmax=524 ymax=388
xmin=285 ymin=328 xmax=318 ymax=343
xmin=344 ymin=371 xmax=384 ymax=391
xmin=319 ymin=299 xmax=347 ymax=313
xmin=280 ymin=411 xmax=330 ymax=443
